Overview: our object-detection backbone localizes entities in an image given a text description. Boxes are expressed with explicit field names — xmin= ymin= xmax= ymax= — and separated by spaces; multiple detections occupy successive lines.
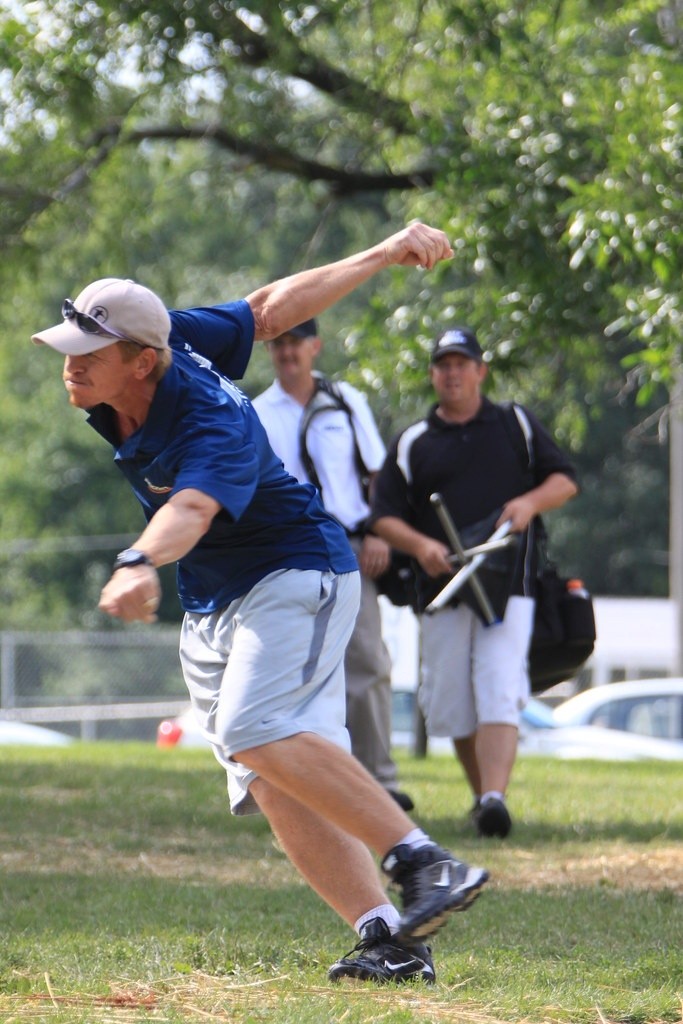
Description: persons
xmin=250 ymin=316 xmax=417 ymax=815
xmin=367 ymin=325 xmax=580 ymax=840
xmin=32 ymin=219 xmax=489 ymax=989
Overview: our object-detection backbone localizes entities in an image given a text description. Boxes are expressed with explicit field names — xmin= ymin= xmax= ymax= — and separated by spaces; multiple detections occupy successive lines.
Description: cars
xmin=154 ymin=651 xmax=563 ymax=754
xmin=545 ymin=675 xmax=683 ymax=765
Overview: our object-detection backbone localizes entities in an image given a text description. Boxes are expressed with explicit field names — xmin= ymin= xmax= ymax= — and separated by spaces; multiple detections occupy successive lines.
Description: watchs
xmin=114 ymin=549 xmax=154 ymax=573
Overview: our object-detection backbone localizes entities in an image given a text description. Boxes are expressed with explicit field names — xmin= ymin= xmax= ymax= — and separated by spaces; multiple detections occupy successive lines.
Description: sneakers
xmin=380 ymin=843 xmax=490 ymax=943
xmin=328 ymin=917 xmax=436 ymax=986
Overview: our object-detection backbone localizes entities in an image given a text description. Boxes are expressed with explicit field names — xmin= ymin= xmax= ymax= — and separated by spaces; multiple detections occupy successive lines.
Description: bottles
xmin=566 ymin=579 xmax=591 ymax=599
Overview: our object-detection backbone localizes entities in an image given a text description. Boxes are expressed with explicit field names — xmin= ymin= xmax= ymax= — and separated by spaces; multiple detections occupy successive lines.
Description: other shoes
xmin=387 ymin=788 xmax=414 ymax=812
xmin=461 ymin=801 xmax=483 ymax=836
xmin=472 ymin=797 xmax=512 ymax=839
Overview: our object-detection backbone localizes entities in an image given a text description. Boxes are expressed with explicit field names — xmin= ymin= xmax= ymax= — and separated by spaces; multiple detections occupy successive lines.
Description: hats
xmin=263 ymin=318 xmax=317 ymax=343
xmin=30 ymin=277 xmax=172 ymax=354
xmin=431 ymin=330 xmax=486 ymax=363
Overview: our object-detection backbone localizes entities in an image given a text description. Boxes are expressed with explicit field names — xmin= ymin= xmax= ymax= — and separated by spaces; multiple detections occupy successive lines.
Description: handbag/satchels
xmin=528 ymin=565 xmax=595 ymax=693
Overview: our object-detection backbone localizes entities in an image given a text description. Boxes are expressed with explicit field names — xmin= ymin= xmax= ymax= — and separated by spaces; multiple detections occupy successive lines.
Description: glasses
xmin=61 ymin=298 xmax=146 ymax=349
